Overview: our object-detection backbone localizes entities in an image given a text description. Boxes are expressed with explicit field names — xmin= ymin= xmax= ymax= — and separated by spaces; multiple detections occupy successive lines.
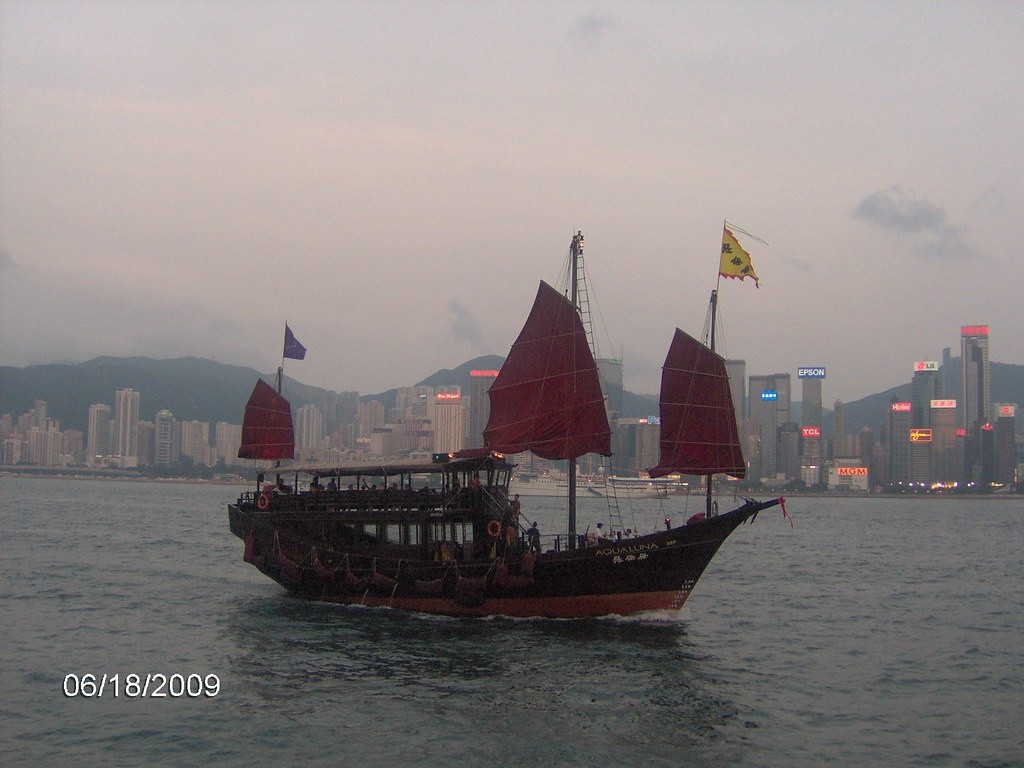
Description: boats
xmin=226 ymin=219 xmax=795 ymax=620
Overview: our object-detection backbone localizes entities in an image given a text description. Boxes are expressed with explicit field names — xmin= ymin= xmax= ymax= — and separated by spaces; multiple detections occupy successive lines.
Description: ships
xmin=476 ymin=467 xmax=677 ymax=498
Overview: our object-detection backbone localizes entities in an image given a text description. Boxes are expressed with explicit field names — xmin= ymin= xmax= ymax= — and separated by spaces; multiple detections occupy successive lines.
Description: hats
xmin=474 ymin=474 xmax=479 ymax=477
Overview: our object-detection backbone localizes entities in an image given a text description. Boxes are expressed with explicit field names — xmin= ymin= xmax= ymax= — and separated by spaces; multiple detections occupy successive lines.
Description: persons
xmin=269 ymin=477 xmax=636 ymax=556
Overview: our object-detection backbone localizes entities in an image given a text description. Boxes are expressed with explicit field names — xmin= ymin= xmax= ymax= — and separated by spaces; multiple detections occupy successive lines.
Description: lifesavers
xmin=487 ymin=519 xmax=503 ymax=537
xmin=258 ymin=495 xmax=269 ymax=511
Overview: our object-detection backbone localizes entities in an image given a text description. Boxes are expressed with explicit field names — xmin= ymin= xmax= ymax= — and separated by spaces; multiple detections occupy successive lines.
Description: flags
xmin=283 ymin=325 xmax=307 ymax=360
